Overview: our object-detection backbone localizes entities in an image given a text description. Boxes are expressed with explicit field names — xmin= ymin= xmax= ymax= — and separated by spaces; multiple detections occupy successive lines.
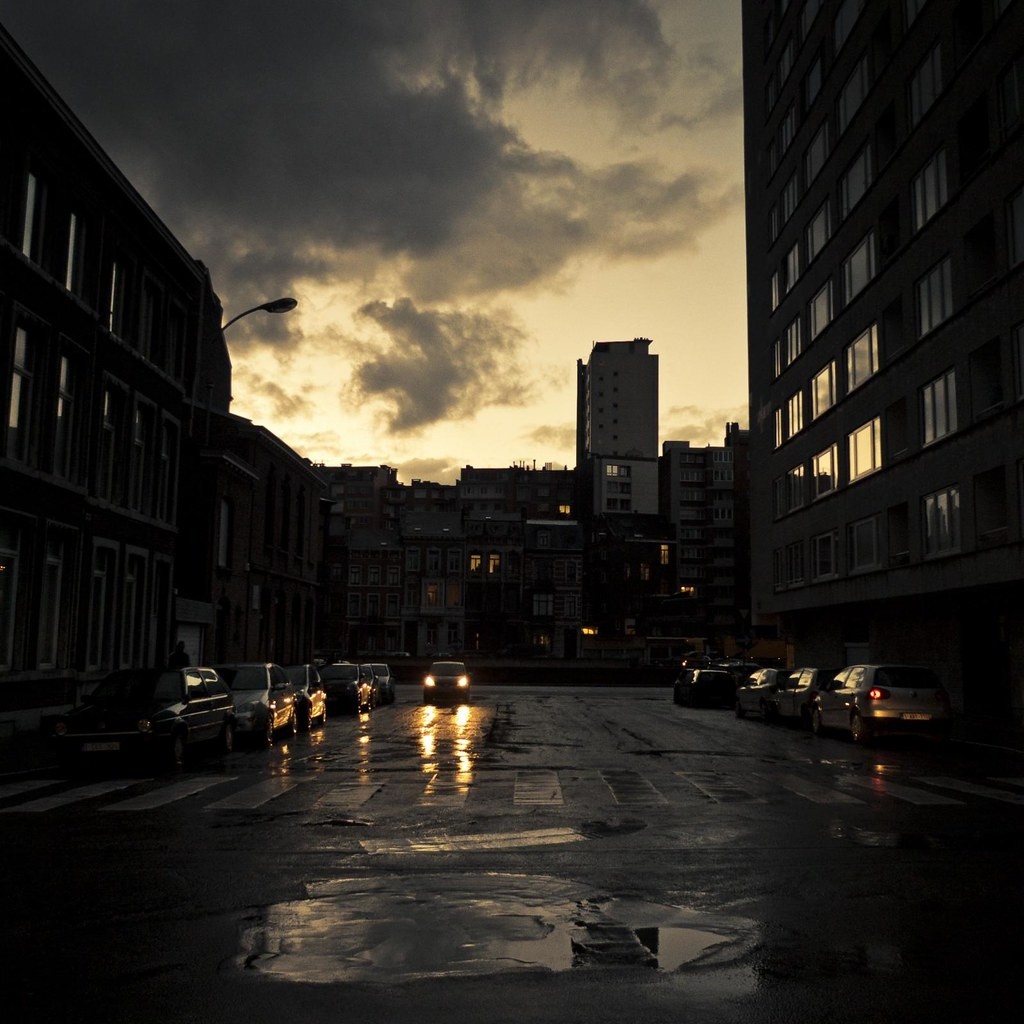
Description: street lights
xmin=163 ymin=295 xmax=299 ymax=672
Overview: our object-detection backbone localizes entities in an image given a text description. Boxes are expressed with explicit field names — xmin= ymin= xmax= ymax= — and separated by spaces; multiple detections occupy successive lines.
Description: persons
xmin=168 ymin=640 xmax=190 ymax=668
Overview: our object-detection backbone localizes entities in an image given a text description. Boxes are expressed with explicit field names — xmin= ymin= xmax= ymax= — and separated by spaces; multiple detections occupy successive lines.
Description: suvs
xmin=814 ymin=662 xmax=951 ymax=745
xmin=673 ymin=668 xmax=737 ymax=710
xmin=772 ymin=666 xmax=840 ymax=723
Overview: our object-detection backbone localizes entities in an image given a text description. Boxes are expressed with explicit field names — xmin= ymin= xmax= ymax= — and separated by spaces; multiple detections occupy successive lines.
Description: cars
xmin=734 ymin=666 xmax=798 ymax=720
xmin=211 ymin=664 xmax=299 ymax=744
xmin=363 ymin=663 xmax=396 ymax=704
xmin=362 ymin=664 xmax=383 ymax=707
xmin=50 ymin=667 xmax=238 ymax=764
xmin=281 ymin=666 xmax=329 ymax=730
xmin=317 ymin=661 xmax=374 ymax=716
xmin=421 ymin=660 xmax=473 ymax=703
